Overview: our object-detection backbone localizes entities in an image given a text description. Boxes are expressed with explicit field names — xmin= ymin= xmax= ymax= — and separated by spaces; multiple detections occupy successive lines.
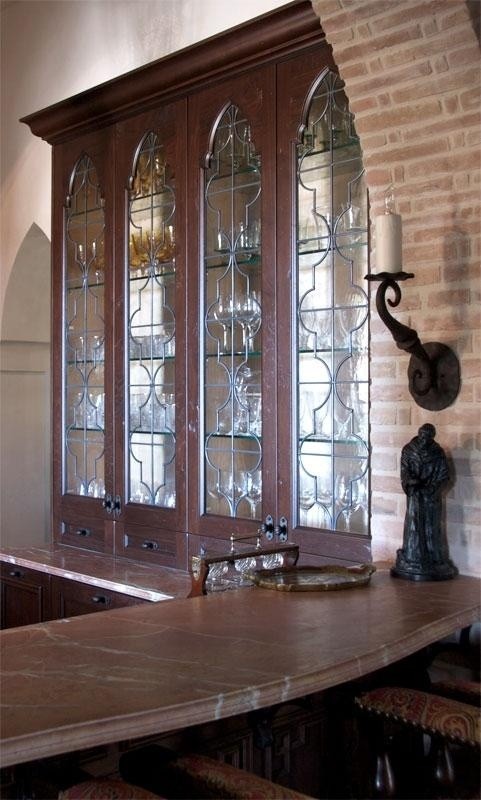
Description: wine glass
xmin=205 ymin=552 xmax=284 ymax=592
xmin=62 ymin=101 xmax=368 ymax=534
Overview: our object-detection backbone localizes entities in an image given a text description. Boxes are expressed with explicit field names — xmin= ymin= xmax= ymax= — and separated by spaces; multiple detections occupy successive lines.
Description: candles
xmin=373 ymin=208 xmax=402 ymax=273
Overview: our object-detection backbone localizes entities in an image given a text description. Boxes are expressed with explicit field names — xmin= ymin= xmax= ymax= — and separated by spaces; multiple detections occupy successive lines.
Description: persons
xmin=395 ymin=424 xmax=453 ymax=575
